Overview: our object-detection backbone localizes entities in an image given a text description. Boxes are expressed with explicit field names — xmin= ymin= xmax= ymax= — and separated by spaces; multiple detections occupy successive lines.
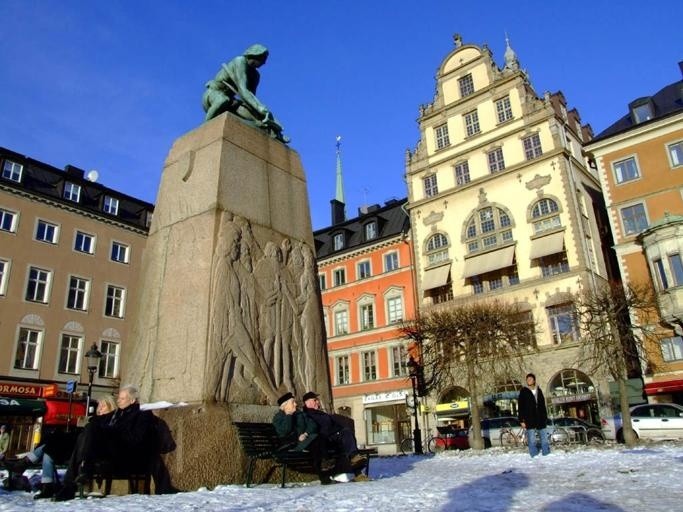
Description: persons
xmin=50 ymin=385 xmax=142 ymax=503
xmin=0 ymin=424 xmax=10 ymax=458
xmin=271 ymin=392 xmax=338 ymax=486
xmin=203 ymin=209 xmax=323 ymax=404
xmin=201 ymin=42 xmax=273 ymax=130
xmin=515 ymin=372 xmax=550 ymax=459
xmin=301 ymin=391 xmax=371 ymax=482
xmin=1 ymin=394 xmax=117 ymax=500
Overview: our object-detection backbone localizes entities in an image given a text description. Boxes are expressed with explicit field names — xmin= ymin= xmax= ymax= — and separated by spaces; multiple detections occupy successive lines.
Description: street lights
xmin=83 ymin=342 xmax=103 ymax=419
xmin=404 ymin=354 xmax=420 ymax=430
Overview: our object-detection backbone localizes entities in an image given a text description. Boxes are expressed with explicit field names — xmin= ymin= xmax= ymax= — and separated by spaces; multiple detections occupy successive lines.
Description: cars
xmin=433 ymin=416 xmax=605 ymax=447
xmin=599 ymin=402 xmax=682 ymax=444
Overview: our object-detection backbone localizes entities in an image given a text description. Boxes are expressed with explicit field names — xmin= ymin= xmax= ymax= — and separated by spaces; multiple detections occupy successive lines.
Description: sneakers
xmin=320 ymin=459 xmax=336 ymax=471
xmin=354 ymin=474 xmax=367 ymax=481
xmin=350 ymin=454 xmax=366 ymax=464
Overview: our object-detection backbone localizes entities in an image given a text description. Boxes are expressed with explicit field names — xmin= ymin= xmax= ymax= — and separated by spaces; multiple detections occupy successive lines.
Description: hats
xmin=303 ymin=392 xmax=320 ymax=402
xmin=278 ymin=392 xmax=295 ymax=406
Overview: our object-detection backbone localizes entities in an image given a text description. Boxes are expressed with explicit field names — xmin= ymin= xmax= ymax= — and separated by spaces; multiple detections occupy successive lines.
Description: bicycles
xmin=398 ymin=428 xmax=447 ymax=456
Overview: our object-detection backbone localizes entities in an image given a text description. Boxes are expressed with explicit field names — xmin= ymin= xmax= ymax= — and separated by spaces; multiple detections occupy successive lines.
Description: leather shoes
xmin=3 ymin=457 xmax=29 ymax=477
xmin=33 ymin=486 xmax=53 ymax=498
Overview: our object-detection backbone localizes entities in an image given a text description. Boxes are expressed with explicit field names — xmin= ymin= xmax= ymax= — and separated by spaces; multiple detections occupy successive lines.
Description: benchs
xmin=231 ymin=422 xmax=378 ymax=488
